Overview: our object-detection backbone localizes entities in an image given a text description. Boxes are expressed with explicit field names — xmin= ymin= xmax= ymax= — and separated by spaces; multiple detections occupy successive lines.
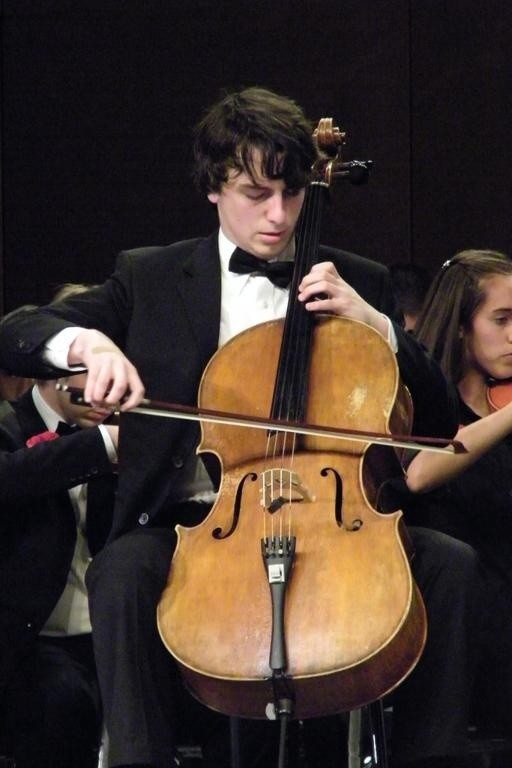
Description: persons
xmin=384 ymin=258 xmax=428 ymax=335
xmin=0 ymin=281 xmax=125 ymax=768
xmin=405 ymin=243 xmax=512 ymax=765
xmin=1 ymin=85 xmax=463 ymax=767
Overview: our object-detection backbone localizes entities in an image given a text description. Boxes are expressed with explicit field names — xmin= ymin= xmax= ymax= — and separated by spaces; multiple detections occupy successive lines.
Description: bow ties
xmin=224 ymin=244 xmax=300 ymax=288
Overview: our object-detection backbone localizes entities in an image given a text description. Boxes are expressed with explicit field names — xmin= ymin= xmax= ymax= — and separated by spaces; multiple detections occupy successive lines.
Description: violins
xmin=486 ymin=377 xmax=511 ymax=416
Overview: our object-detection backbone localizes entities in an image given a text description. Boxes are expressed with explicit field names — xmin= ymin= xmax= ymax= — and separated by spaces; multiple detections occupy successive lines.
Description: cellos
xmin=155 ymin=118 xmax=426 ymax=724
